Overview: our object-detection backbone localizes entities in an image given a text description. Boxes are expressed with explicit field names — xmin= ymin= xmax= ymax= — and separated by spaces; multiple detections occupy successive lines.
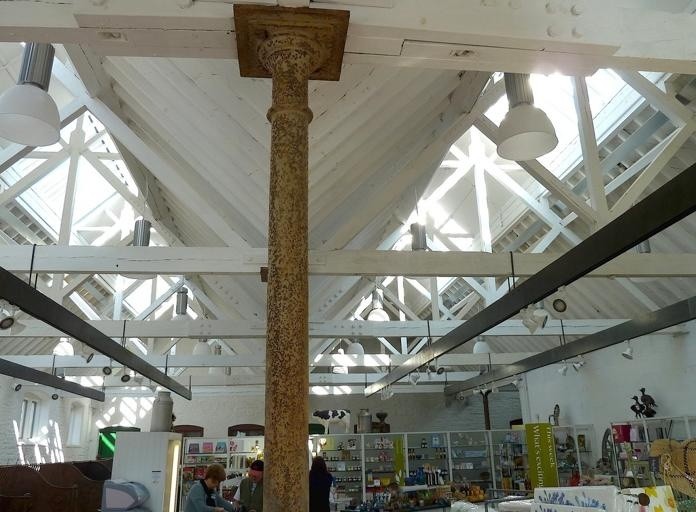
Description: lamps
xmin=172 ymin=286 xmax=227 ymax=376
xmin=0 ymin=40 xmax=61 ymax=147
xmin=0 ymin=299 xmax=159 ymax=399
xmin=495 ymin=69 xmax=560 ymax=161
xmin=120 ymin=167 xmax=161 ymax=281
xmin=333 ymin=283 xmax=394 ymax=373
xmin=400 ymin=184 xmax=437 ymax=280
xmin=407 ymin=300 xmax=636 ymax=402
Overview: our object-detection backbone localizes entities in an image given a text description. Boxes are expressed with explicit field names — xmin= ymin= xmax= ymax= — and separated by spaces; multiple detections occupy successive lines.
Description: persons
xmin=309 ymin=455 xmax=333 ymax=511
xmin=183 ymin=464 xmax=234 ymax=511
xmin=232 ymin=459 xmax=263 ymax=511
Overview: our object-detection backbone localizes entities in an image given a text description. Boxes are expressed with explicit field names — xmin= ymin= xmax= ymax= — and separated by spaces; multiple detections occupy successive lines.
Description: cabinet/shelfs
xmin=364 ymin=433 xmax=406 ymax=492
xmin=182 ymin=436 xmax=264 ymax=502
xmin=610 ymin=416 xmax=696 ymax=489
xmin=553 ymin=428 xmax=593 ymax=486
xmin=310 ymin=434 xmax=364 ymax=508
xmin=491 ymin=430 xmax=533 ymax=495
xmin=407 ymin=431 xmax=449 ymax=488
xmin=450 ymin=430 xmax=495 ymax=488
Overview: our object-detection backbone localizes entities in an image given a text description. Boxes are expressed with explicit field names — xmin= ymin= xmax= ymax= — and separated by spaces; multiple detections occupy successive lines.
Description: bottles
xmin=356 ymin=407 xmax=373 ymax=434
xmin=150 ymin=390 xmax=174 ymax=432
